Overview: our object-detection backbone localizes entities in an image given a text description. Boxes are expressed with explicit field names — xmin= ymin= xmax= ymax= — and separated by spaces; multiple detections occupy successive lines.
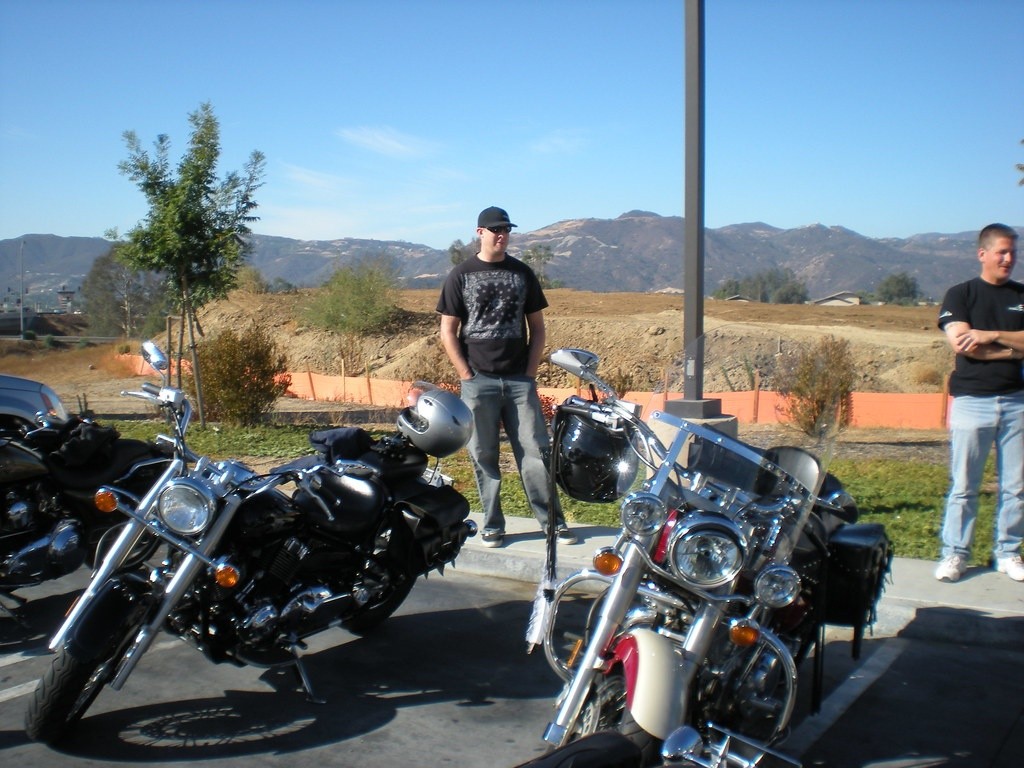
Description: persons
xmin=437 ymin=206 xmax=577 ymax=549
xmin=933 ymin=223 xmax=1024 ymax=583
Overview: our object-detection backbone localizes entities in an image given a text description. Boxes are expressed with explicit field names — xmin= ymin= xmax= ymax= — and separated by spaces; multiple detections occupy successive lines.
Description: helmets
xmin=539 ymin=412 xmax=639 ymax=504
xmin=396 ymin=381 xmax=473 ymax=458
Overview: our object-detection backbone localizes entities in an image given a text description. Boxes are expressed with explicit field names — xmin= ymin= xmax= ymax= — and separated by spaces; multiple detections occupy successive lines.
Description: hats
xmin=478 ymin=206 xmax=518 ymax=228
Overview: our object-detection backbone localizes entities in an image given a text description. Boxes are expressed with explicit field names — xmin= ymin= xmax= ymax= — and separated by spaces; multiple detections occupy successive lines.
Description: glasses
xmin=483 ymin=227 xmax=511 ymax=233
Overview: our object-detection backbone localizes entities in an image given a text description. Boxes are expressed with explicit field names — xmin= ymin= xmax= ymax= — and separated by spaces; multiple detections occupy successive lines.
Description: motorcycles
xmin=23 ymin=341 xmax=476 ymax=748
xmin=0 ymin=393 xmax=178 ymax=617
xmin=514 ymin=349 xmax=895 ymax=767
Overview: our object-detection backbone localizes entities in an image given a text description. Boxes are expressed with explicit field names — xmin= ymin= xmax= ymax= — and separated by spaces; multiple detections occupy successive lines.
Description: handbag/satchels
xmin=822 ymin=523 xmax=894 ymax=660
xmin=392 ymin=484 xmax=469 ymax=577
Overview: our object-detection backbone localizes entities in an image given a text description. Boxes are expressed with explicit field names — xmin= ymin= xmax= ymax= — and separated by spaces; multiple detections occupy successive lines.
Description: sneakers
xmin=556 ymin=529 xmax=578 ymax=545
xmin=992 ymin=556 xmax=1024 ymax=581
xmin=935 ymin=554 xmax=966 ymax=583
xmin=481 ymin=532 xmax=503 ymax=547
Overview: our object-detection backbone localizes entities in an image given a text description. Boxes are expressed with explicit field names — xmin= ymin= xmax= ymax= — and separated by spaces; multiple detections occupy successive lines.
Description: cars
xmin=0 ymin=374 xmax=69 ymax=448
